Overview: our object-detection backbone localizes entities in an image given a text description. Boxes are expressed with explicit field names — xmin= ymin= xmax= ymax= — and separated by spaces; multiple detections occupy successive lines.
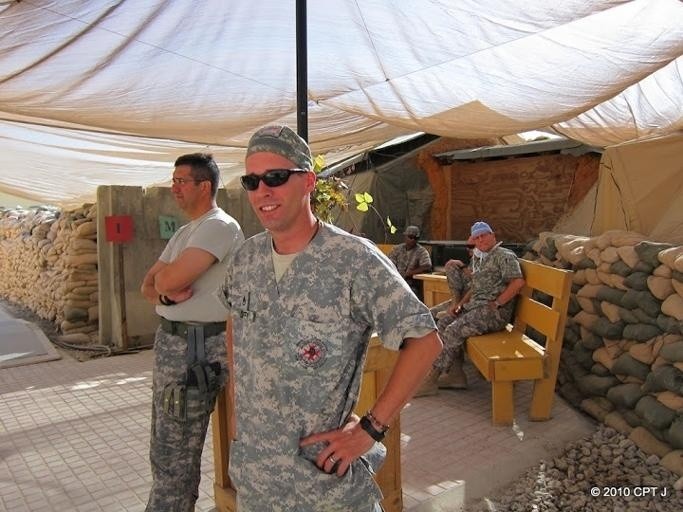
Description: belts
xmin=159 ymin=315 xmax=228 ymax=339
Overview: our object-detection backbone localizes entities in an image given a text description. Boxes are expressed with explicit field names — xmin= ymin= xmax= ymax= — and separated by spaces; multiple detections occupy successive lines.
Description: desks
xmin=412 ymin=272 xmax=451 ymax=306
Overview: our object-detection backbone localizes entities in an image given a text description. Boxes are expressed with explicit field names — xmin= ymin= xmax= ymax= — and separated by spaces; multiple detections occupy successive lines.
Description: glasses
xmin=238 ymin=169 xmax=311 ymax=191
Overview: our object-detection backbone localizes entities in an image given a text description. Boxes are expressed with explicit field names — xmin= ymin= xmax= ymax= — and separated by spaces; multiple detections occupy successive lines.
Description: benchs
xmin=456 ymin=257 xmax=575 ymax=424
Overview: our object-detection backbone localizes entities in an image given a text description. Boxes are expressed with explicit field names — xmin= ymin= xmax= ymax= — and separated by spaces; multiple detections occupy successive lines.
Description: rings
xmin=328 ymin=456 xmax=337 ymax=464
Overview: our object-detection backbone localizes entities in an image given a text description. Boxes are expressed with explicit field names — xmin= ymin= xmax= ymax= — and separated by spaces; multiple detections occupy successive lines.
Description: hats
xmin=245 ymin=125 xmax=313 ymax=173
xmin=402 ymin=226 xmax=420 ymax=236
xmin=470 ymin=221 xmax=492 ymax=240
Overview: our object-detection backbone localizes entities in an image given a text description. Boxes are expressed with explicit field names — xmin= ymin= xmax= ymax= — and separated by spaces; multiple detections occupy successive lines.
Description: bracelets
xmin=367 ymin=412 xmax=391 ymax=433
xmin=359 ymin=414 xmax=385 ymax=442
xmin=159 ymin=294 xmax=168 ymax=306
xmin=163 ymin=293 xmax=177 ymax=305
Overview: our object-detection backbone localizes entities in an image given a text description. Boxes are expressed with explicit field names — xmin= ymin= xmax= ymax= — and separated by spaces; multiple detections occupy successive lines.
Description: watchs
xmin=494 ymin=300 xmax=502 ymax=310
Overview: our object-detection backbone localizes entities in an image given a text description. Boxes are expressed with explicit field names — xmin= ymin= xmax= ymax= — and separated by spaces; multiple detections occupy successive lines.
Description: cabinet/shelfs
xmin=212 ymin=330 xmax=403 ymax=512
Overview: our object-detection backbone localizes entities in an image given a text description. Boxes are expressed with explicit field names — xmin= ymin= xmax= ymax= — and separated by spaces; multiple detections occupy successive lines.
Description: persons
xmin=411 ymin=222 xmax=526 ymax=398
xmin=430 ymin=234 xmax=481 ymax=323
xmin=388 ymin=225 xmax=432 ymax=302
xmin=214 ymin=124 xmax=443 ymax=512
xmin=140 ymin=152 xmax=248 ymax=512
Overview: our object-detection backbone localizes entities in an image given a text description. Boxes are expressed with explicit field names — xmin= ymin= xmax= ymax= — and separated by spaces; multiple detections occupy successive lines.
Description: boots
xmin=410 ymin=368 xmax=440 ymax=398
xmin=438 ymin=359 xmax=468 ymax=390
